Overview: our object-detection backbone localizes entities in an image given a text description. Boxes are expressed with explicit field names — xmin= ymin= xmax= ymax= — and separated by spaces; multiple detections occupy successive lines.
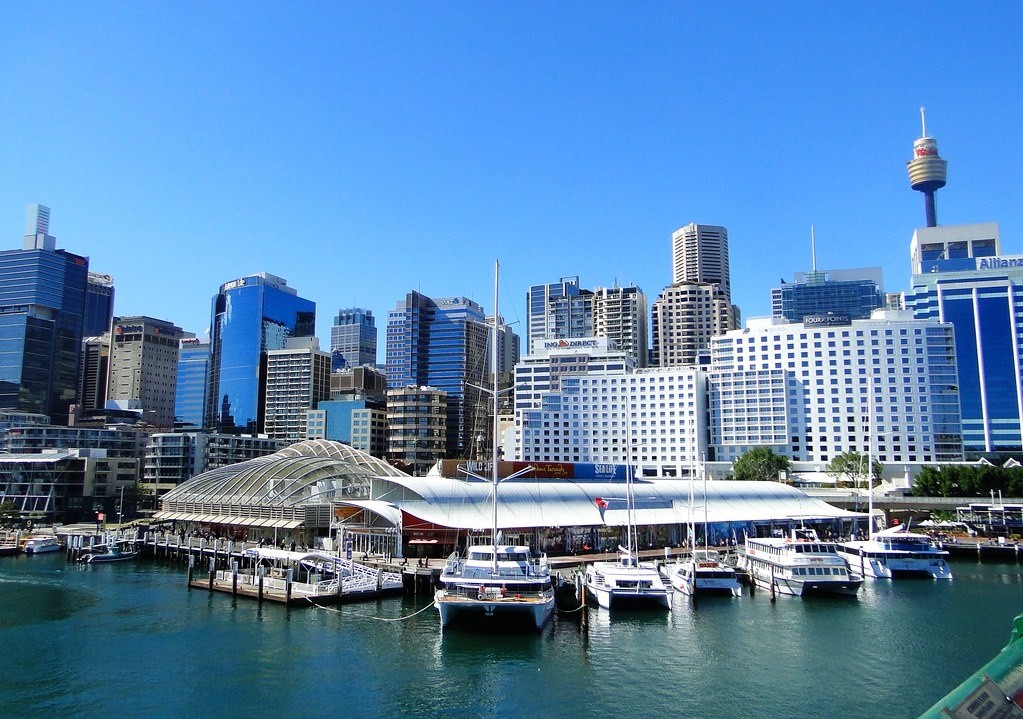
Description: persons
xmin=402 ymin=557 xmax=407 ymax=566
xmin=155 ymin=524 xmax=310 ymax=552
xmin=987 ymin=537 xmax=992 ymax=545
xmin=826 ymin=528 xmax=866 ymax=541
xmin=363 ymin=552 xmax=368 ymax=561
xmin=425 ymin=555 xmax=428 ymax=568
xmin=419 ymin=558 xmax=423 ymax=568
xmin=948 ymin=539 xmax=953 ymax=544
xmin=586 ymin=536 xmax=736 ymax=555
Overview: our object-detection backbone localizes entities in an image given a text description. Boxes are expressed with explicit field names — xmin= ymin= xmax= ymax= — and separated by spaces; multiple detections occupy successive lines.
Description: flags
xmin=595 ymin=496 xmax=609 ymax=522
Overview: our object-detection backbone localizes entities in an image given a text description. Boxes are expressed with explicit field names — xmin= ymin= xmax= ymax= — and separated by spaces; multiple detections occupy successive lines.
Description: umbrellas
xmin=917 ymin=519 xmax=962 ymax=528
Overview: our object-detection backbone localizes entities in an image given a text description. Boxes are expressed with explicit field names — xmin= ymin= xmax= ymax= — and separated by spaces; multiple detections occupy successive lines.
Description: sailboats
xmin=570 ymin=396 xmax=675 ymax=610
xmin=659 ymin=418 xmax=742 ymax=597
xmin=433 ymin=258 xmax=556 ymax=631
xmin=837 ymin=373 xmax=955 ymax=585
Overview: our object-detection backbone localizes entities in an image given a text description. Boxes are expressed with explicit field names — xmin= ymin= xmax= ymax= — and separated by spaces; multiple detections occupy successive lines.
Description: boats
xmin=732 ymin=500 xmax=865 ymax=598
xmin=22 ymin=537 xmax=62 ymax=553
xmin=81 ymin=546 xmax=140 ymax=564
